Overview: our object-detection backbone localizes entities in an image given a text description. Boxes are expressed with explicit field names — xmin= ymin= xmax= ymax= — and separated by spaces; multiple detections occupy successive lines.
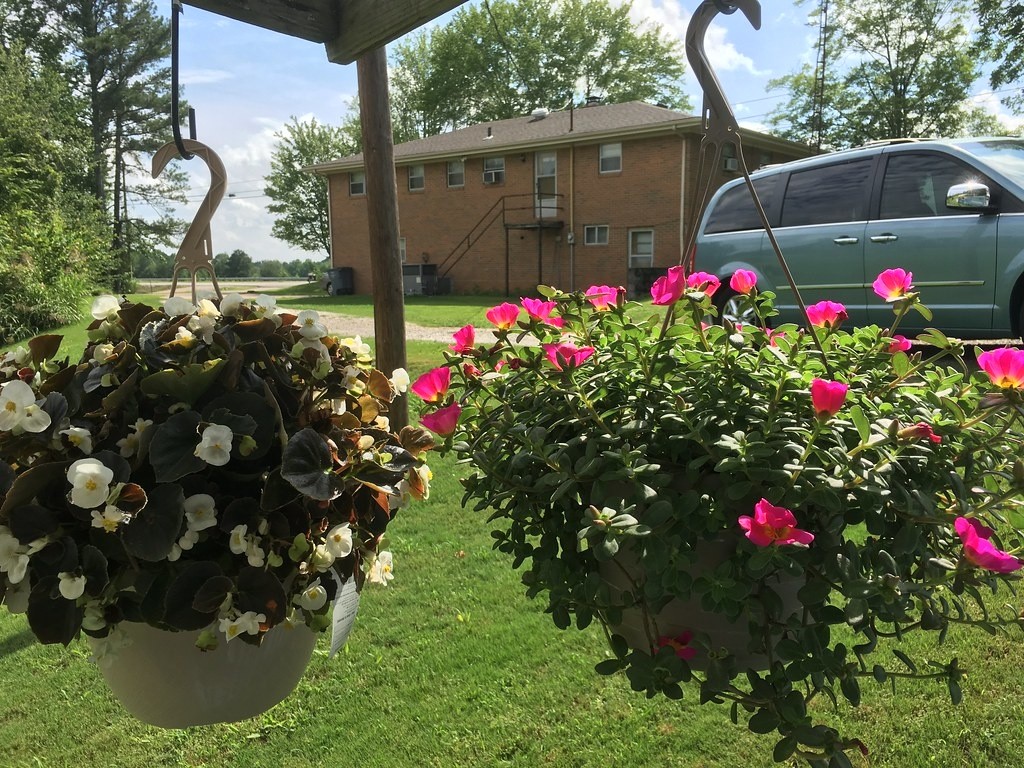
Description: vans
xmin=687 ymin=135 xmax=1024 ymax=355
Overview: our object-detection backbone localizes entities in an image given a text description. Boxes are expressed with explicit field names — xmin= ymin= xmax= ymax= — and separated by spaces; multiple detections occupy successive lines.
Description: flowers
xmin=404 ymin=265 xmax=1024 ymax=768
xmin=2 ymin=294 xmax=440 ymax=651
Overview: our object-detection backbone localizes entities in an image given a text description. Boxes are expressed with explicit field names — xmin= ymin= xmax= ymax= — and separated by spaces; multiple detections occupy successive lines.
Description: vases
xmin=579 ymin=520 xmax=827 ymax=684
xmin=83 ymin=611 xmax=320 ymax=727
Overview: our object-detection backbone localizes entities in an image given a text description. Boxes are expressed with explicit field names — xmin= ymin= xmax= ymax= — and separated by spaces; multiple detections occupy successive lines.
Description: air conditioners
xmin=482 ymin=170 xmax=499 ymax=184
xmin=722 ymin=158 xmax=738 ymax=172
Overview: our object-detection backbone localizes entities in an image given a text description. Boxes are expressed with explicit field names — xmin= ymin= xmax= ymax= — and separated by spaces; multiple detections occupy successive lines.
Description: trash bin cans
xmin=328 ymin=265 xmax=355 ymax=297
